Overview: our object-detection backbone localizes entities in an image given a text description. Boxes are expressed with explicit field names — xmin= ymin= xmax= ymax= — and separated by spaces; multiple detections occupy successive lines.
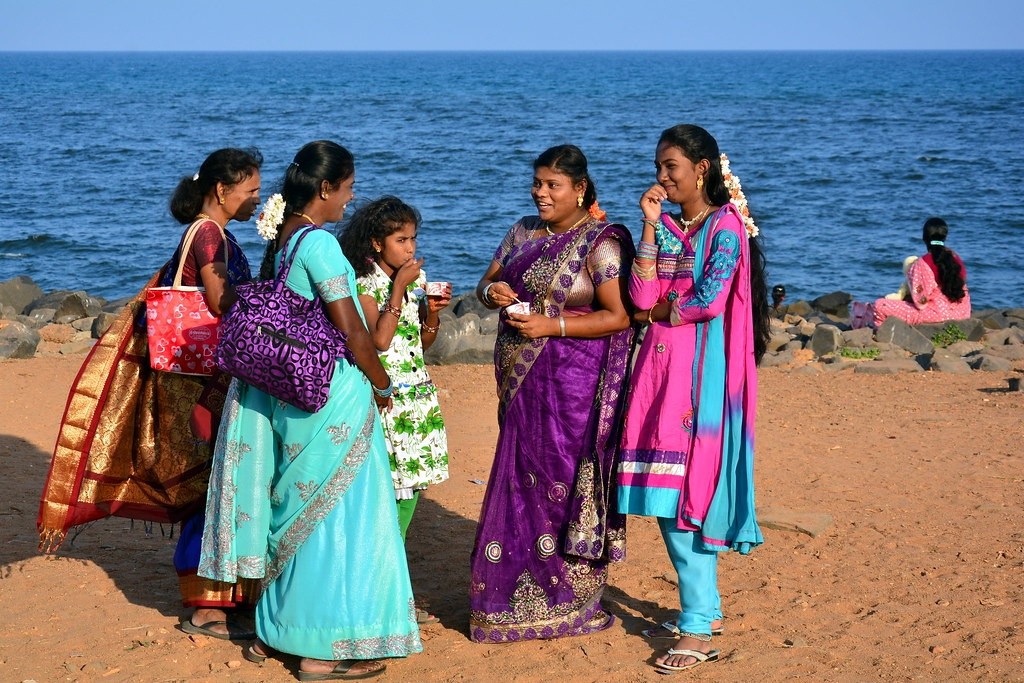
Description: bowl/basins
xmin=425 ymin=282 xmax=448 ymax=296
xmin=506 ymin=302 xmax=531 ymax=320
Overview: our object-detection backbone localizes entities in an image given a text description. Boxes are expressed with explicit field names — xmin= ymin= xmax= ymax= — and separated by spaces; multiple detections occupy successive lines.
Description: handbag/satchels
xmin=216 ymin=222 xmax=347 ymax=414
xmin=143 ymin=219 xmax=239 ymax=377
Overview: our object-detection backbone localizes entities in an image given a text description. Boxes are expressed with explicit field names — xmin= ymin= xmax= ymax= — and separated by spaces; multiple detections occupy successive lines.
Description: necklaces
xmin=538 ymin=211 xmax=592 ymax=238
xmin=678 ymin=200 xmax=711 ymax=261
xmin=194 ymin=214 xmax=243 ymax=250
xmin=293 ymin=212 xmax=315 ymax=224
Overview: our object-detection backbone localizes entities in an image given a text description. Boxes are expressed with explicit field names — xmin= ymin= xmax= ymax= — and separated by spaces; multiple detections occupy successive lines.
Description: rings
xmin=519 ymin=322 xmax=525 ymax=330
xmin=491 ymin=293 xmax=498 ymax=300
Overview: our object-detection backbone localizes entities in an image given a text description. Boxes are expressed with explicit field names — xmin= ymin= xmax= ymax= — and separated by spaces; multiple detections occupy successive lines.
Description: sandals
xmin=417 ymin=610 xmax=439 ymax=625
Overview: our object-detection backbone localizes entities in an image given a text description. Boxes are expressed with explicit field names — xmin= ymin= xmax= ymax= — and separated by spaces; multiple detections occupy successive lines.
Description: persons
xmin=872 ymin=218 xmax=970 ymax=336
xmin=196 ymin=140 xmax=423 ymax=681
xmin=469 ymin=144 xmax=640 ymax=646
xmin=154 ymin=147 xmax=262 ymax=641
xmin=617 ymin=123 xmax=773 ymax=669
xmin=334 ymin=195 xmax=450 ymax=545
xmin=770 ymin=285 xmax=785 ymax=308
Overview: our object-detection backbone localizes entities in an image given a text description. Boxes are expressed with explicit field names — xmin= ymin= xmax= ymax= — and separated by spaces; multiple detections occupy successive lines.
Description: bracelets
xmin=641 ymin=218 xmax=660 ymax=231
xmin=482 ymin=281 xmax=509 ymax=307
xmin=559 ymin=316 xmax=565 ymax=337
xmin=423 ymin=315 xmax=441 ymax=333
xmin=372 ymin=376 xmax=393 ymax=398
xmin=383 ymin=304 xmax=402 ymax=320
xmin=648 ymin=303 xmax=659 ymax=324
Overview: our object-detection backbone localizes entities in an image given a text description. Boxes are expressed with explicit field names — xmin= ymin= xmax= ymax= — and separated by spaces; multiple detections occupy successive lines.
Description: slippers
xmin=246 ymin=647 xmax=272 ymax=663
xmin=298 ymin=659 xmax=388 ymax=681
xmin=642 ymin=618 xmax=725 ymax=639
xmin=181 ymin=614 xmax=256 ymax=641
xmin=655 ymin=648 xmax=720 ymax=671
